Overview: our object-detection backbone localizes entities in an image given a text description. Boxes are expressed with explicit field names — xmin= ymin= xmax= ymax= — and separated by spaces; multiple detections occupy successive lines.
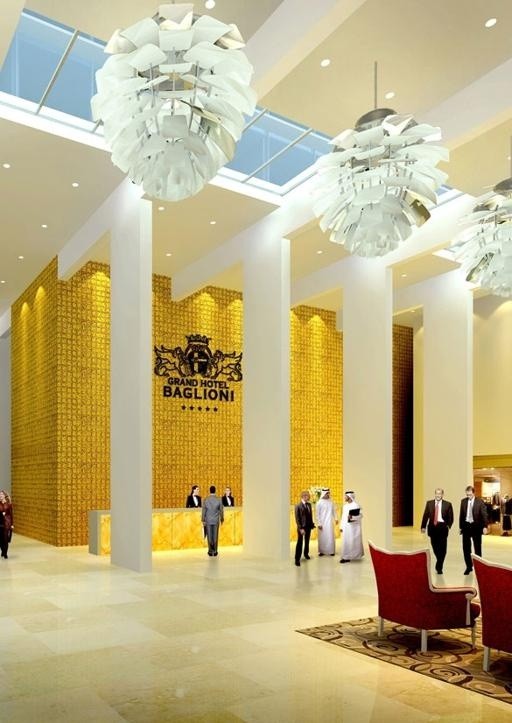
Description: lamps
xmin=457 ymin=135 xmax=512 ymax=300
xmin=89 ymin=0 xmax=258 ymax=205
xmin=308 ymin=60 xmax=452 ymax=259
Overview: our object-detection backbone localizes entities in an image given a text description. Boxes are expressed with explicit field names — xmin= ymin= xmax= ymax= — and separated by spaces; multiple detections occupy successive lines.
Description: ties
xmin=432 ymin=501 xmax=439 ymax=526
xmin=468 ymin=499 xmax=474 ymax=524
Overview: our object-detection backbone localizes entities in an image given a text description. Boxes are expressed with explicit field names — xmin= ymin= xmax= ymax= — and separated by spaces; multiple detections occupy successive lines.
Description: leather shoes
xmin=340 ymin=559 xmax=351 ymax=563
xmin=295 ymin=556 xmax=301 ymax=566
xmin=463 ymin=567 xmax=473 ymax=575
xmin=305 ymin=554 xmax=311 ymax=559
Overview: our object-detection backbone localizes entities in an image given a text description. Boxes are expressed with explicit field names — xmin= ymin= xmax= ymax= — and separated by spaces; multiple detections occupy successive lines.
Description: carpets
xmin=295 ymin=598 xmax=512 ymax=705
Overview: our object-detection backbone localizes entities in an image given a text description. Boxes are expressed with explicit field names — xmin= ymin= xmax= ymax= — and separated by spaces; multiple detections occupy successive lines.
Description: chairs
xmin=469 ymin=552 xmax=512 ymax=671
xmin=367 ymin=539 xmax=481 ymax=655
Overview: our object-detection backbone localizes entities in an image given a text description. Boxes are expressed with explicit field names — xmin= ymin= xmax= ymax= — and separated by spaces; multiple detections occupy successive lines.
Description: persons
xmin=221 ymin=486 xmax=234 ymax=506
xmin=0 ymin=489 xmax=14 ymax=558
xmin=315 ymin=487 xmax=339 ymax=556
xmin=340 ymin=489 xmax=364 ymax=563
xmin=201 ymin=485 xmax=224 ymax=555
xmin=421 ymin=488 xmax=453 ymax=573
xmin=185 ymin=484 xmax=201 ymax=506
xmin=500 ymin=492 xmax=511 ymax=535
xmin=458 ymin=486 xmax=488 ymax=575
xmin=294 ymin=490 xmax=315 ymax=565
